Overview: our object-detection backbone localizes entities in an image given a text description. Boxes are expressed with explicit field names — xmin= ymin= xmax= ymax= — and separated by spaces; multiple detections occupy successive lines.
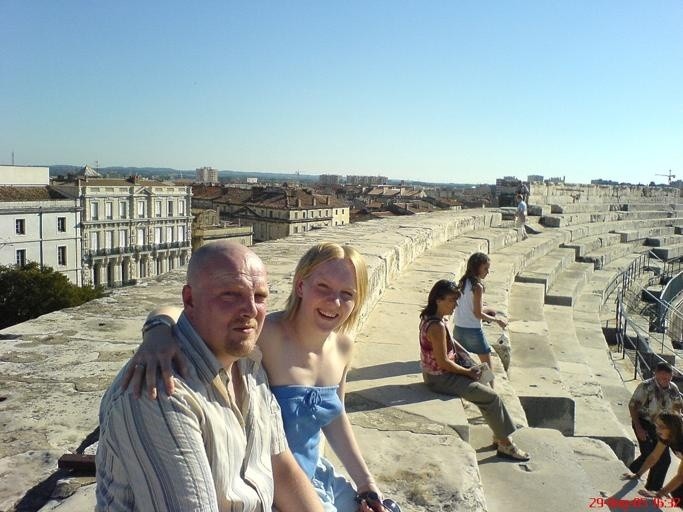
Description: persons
xmin=95 ymin=241 xmax=324 ymax=512
xmin=628 ymin=361 xmax=683 ymax=491
xmin=420 ymin=280 xmax=531 ymax=460
xmin=515 ymin=194 xmax=528 ymax=240
xmin=120 ymin=245 xmax=389 ymax=512
xmin=452 ymin=252 xmax=507 ymax=370
xmin=621 ymin=409 xmax=683 ymax=509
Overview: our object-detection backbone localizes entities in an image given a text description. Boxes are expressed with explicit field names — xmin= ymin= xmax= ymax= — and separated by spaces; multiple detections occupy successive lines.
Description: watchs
xmin=143 ymin=318 xmax=171 ymax=334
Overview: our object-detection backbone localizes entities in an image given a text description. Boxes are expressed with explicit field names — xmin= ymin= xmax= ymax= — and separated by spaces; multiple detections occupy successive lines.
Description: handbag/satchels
xmin=493 ymin=335 xmax=512 ymax=372
xmin=452 ymin=337 xmax=478 ymax=367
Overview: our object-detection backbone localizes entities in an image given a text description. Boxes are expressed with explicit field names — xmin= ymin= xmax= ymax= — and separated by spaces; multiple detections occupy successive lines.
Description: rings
xmin=135 ymin=364 xmax=144 ymax=369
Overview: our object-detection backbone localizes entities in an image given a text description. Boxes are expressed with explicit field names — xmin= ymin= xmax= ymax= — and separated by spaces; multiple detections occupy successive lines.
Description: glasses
xmin=354 ymin=491 xmax=401 ymax=512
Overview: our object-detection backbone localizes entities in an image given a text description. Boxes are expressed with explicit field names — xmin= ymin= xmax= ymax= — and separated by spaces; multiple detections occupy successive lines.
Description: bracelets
xmin=357 ymin=479 xmax=375 ymax=493
xmin=636 ymin=474 xmax=640 ymax=479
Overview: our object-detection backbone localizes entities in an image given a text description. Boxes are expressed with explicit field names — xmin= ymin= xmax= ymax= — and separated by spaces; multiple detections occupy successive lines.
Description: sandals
xmin=493 ymin=436 xmax=530 ymax=461
